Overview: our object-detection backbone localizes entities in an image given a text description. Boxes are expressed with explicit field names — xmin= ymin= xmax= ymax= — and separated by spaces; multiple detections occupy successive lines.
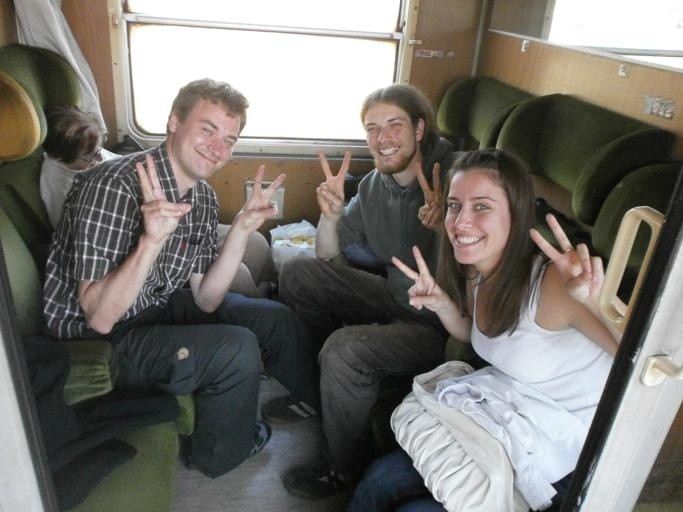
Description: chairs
xmin=422 ymin=76 xmax=538 ymax=154
xmin=0 ymin=41 xmax=184 ymax=512
xmin=442 ymin=90 xmax=683 ymax=453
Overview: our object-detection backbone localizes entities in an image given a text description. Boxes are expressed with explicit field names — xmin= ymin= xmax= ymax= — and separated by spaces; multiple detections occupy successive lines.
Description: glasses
xmin=74 ymin=138 xmax=109 ymax=164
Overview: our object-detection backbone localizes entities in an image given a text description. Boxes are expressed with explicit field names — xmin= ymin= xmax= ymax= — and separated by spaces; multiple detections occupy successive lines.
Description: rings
xmin=561 ymin=247 xmax=574 ymax=253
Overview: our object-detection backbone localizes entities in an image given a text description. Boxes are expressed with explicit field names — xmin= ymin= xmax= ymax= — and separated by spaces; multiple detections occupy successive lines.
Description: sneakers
xmin=283 ymin=460 xmax=354 ymax=499
xmin=250 ymin=420 xmax=272 ymax=457
xmin=261 ymin=393 xmax=323 ymax=425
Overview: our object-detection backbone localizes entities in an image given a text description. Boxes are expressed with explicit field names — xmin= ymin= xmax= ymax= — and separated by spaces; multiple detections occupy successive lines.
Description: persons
xmin=341 ymin=145 xmax=634 ymax=510
xmin=39 ymin=78 xmax=318 ymax=475
xmin=257 ymin=84 xmax=465 ymax=501
xmin=38 ymin=103 xmax=278 ymax=299
